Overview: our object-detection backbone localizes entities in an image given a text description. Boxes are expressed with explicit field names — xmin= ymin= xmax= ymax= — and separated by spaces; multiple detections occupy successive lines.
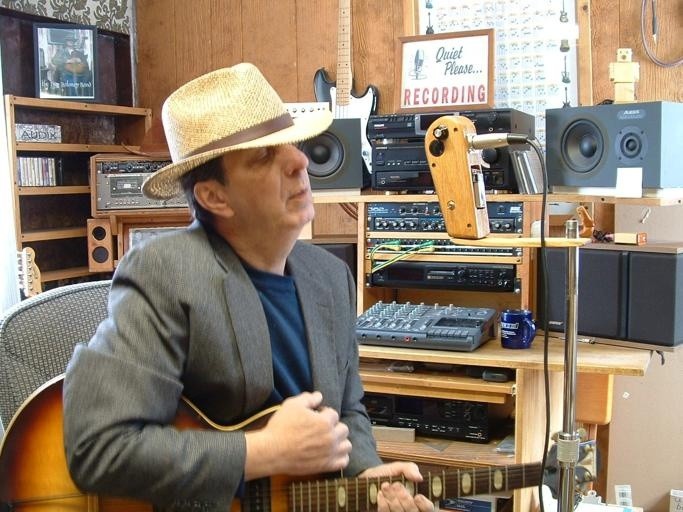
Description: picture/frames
xmin=31 ymin=22 xmax=97 ymax=101
xmin=390 ymin=29 xmax=497 ymax=113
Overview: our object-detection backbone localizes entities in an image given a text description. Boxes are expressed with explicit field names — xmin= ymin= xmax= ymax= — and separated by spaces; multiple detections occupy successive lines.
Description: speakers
xmin=87 ymin=218 xmax=114 ymax=273
xmin=545 ymin=101 xmax=683 ymax=188
xmin=538 ymin=247 xmax=627 ymax=342
xmin=294 ymin=118 xmax=362 ymax=189
xmin=627 ymin=251 xmax=683 ymax=346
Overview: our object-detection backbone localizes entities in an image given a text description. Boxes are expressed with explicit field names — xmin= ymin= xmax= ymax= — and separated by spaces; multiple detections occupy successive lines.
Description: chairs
xmin=1 ymin=276 xmax=109 ymax=428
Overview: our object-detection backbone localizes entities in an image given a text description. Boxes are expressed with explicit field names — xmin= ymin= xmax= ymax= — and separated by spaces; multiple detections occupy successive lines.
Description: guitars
xmin=2 ymin=368 xmax=595 ymax=512
xmin=313 ymin=1 xmax=378 ymax=190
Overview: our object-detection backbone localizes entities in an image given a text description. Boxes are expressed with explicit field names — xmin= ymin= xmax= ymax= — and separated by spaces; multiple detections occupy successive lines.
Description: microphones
xmin=424 ymin=116 xmax=528 ymax=239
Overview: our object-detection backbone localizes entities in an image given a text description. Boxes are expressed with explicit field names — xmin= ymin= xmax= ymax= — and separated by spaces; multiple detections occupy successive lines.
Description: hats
xmin=141 ymin=62 xmax=334 ymax=200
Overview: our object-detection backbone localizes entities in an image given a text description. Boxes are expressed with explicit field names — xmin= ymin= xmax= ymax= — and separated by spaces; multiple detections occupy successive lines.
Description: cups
xmin=500 ymin=309 xmax=537 ymax=349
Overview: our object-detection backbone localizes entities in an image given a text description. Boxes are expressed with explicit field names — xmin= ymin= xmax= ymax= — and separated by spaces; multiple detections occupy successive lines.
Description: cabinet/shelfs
xmin=317 ymin=189 xmax=683 ymax=319
xmin=350 ymin=335 xmax=647 ymax=511
xmin=5 ymin=97 xmax=149 ymax=305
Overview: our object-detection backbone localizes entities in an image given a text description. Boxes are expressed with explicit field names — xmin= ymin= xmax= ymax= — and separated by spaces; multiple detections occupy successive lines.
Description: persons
xmin=60 ymin=60 xmax=435 ymax=512
xmin=51 ymin=35 xmax=89 ymax=83
